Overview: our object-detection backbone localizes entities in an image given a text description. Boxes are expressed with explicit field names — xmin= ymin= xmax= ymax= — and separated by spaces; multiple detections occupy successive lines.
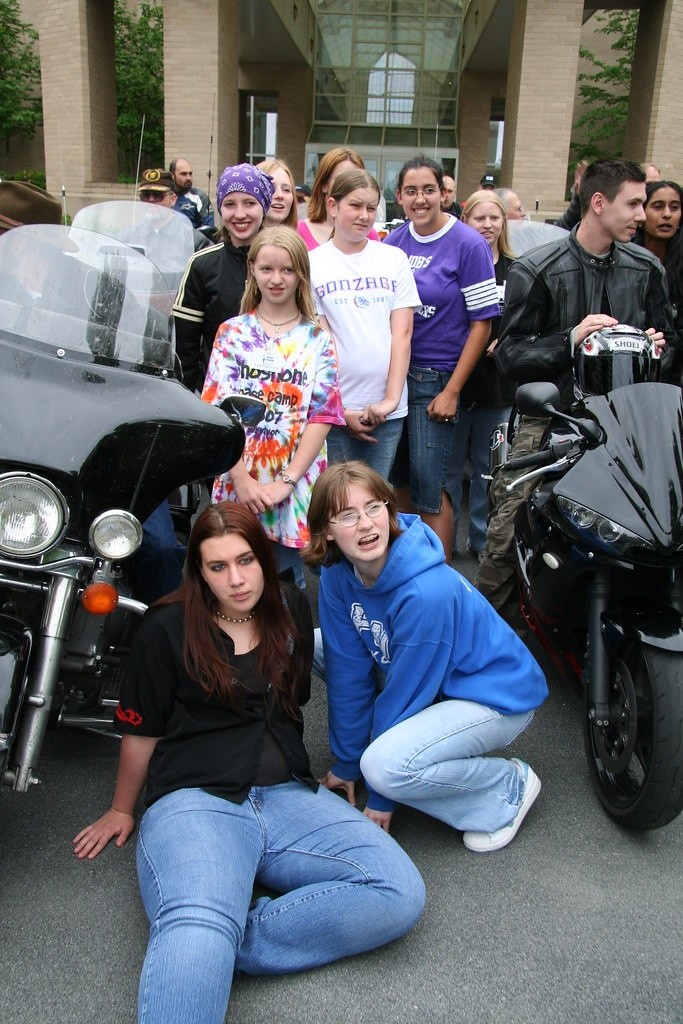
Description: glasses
xmin=139 ymin=190 xmax=173 ymax=203
xmin=400 ymin=184 xmax=443 ymax=198
xmin=327 ymin=499 xmax=388 ymax=527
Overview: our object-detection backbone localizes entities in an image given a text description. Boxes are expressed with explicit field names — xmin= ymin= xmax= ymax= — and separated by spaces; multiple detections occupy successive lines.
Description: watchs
xmin=283 ymin=474 xmax=297 ymax=487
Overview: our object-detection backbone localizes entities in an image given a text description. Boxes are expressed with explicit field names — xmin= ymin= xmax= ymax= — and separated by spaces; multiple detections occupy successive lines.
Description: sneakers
xmin=463 ymin=758 xmax=542 ymax=853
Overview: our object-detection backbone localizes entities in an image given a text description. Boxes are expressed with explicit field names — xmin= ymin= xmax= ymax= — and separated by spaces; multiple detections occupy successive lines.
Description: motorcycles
xmin=1 ymin=204 xmax=268 ymax=794
xmin=482 ymin=381 xmax=683 ymax=833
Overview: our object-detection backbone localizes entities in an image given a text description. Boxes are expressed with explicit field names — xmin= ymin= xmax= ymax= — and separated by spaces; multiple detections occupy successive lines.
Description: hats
xmin=479 ymin=173 xmax=497 ymax=188
xmin=138 ymin=168 xmax=175 ymax=191
xmin=0 ymin=180 xmax=79 ymax=252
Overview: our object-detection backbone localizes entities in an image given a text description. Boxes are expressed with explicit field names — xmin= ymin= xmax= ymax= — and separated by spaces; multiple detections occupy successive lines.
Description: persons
xmin=257 ymin=160 xmax=299 ymax=228
xmin=138 ymin=169 xmax=215 ymax=253
xmin=199 ymin=226 xmax=346 ymax=706
xmin=308 ymin=169 xmax=423 ymax=483
xmin=633 ymin=180 xmax=683 ymax=304
xmin=439 ymin=171 xmax=463 ymax=220
xmin=494 ymin=160 xmax=588 ymax=231
xmin=446 ymin=189 xmax=516 ymax=562
xmin=472 ymin=157 xmax=678 ymax=611
xmin=382 ymin=156 xmax=500 ymax=567
xmin=298 ymin=148 xmax=380 ymax=251
xmin=479 ymin=173 xmax=496 ymax=192
xmin=640 ymin=163 xmax=661 ymax=182
xmin=300 ymin=461 xmax=549 ymax=852
xmin=168 ymin=163 xmax=275 ymax=395
xmin=74 ymin=501 xmax=427 ymax=1024
xmin=170 ymin=157 xmax=215 ymax=228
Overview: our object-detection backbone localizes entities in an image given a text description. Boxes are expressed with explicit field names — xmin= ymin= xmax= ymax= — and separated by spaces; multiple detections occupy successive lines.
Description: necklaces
xmin=216 ymin=612 xmax=255 ymax=623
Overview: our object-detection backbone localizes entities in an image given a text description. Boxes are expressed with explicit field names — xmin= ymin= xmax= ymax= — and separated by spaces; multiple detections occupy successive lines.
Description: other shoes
xmin=466 ymin=546 xmax=487 ymax=562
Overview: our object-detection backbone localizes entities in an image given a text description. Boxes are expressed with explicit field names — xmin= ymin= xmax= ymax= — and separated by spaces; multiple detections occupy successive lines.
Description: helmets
xmin=572 ymin=321 xmax=661 ymax=410
